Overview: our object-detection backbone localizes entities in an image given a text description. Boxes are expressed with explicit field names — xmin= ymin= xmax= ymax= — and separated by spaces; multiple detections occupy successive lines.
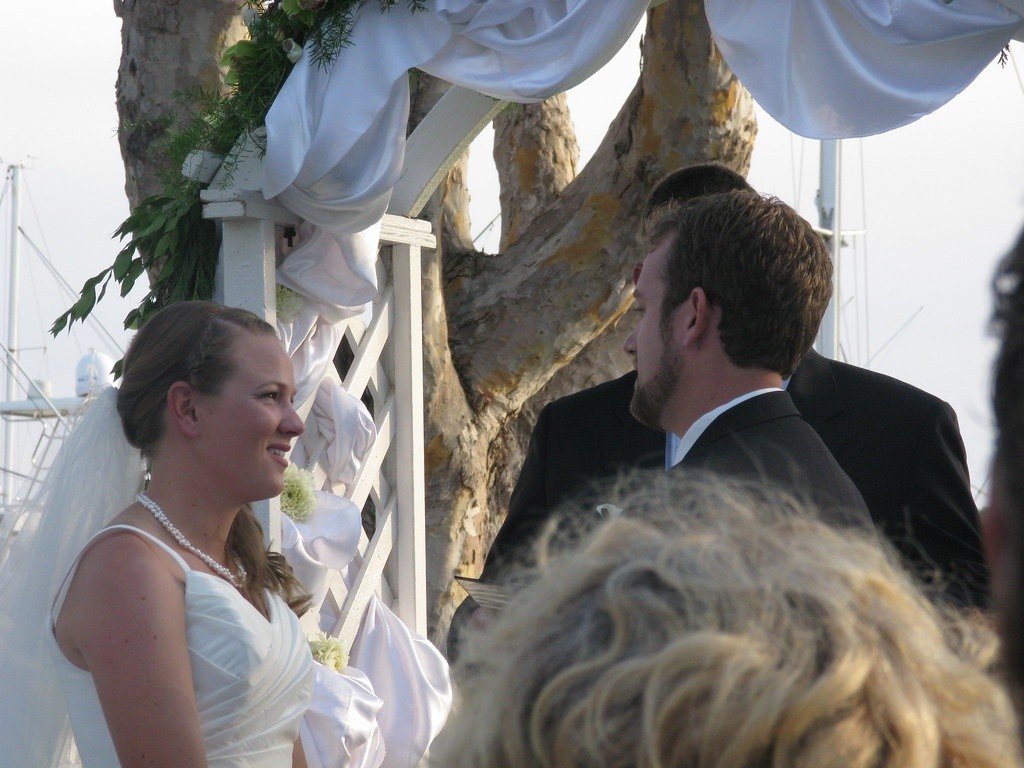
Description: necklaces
xmin=136 ymin=490 xmax=247 ymax=589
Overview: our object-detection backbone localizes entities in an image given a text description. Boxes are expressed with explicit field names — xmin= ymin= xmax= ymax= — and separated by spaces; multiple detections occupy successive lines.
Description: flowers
xmin=280 ymin=461 xmax=317 ymax=521
xmin=308 ymin=629 xmax=351 ymax=670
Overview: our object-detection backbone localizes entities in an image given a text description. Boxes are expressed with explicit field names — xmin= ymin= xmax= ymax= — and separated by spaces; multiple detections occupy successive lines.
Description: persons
xmin=426 ymin=162 xmax=1023 ymax=768
xmin=0 ymin=296 xmax=388 ymax=768
